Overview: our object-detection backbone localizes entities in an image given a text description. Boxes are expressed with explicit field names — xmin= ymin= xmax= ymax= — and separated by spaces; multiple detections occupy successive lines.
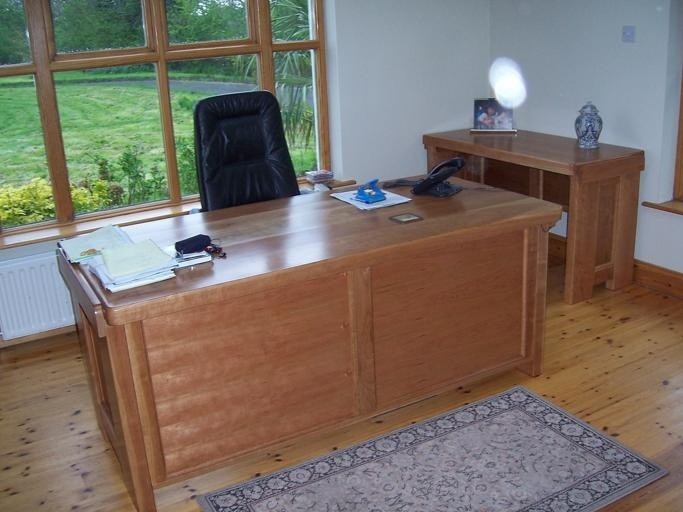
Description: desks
xmin=57 ymin=175 xmax=561 ymax=511
xmin=416 ymin=127 xmax=645 ymax=305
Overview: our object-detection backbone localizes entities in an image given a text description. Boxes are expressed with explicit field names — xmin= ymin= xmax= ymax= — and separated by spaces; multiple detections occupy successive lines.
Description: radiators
xmin=1 ymin=247 xmax=84 ymax=342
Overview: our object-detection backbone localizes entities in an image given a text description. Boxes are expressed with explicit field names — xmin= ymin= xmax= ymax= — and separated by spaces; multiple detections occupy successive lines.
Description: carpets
xmin=194 ymin=380 xmax=671 ymax=511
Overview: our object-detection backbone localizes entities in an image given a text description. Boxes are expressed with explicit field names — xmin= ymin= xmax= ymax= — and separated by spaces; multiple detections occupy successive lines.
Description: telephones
xmin=414 ymin=157 xmax=466 ymax=198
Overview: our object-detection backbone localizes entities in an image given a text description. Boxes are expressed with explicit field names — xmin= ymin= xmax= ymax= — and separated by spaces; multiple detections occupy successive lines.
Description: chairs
xmin=191 ymin=87 xmax=299 ymax=211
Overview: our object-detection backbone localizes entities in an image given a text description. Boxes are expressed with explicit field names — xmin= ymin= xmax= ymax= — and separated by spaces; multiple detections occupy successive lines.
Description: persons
xmin=474 ymin=104 xmax=512 ymax=129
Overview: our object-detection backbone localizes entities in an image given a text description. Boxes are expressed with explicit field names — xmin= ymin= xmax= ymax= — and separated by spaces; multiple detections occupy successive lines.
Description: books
xmin=303 ymin=169 xmax=338 ymax=185
xmin=161 ymin=241 xmax=212 ymax=269
xmin=58 ymin=222 xmax=178 ymax=295
xmin=176 ymin=250 xmax=206 ymax=259
